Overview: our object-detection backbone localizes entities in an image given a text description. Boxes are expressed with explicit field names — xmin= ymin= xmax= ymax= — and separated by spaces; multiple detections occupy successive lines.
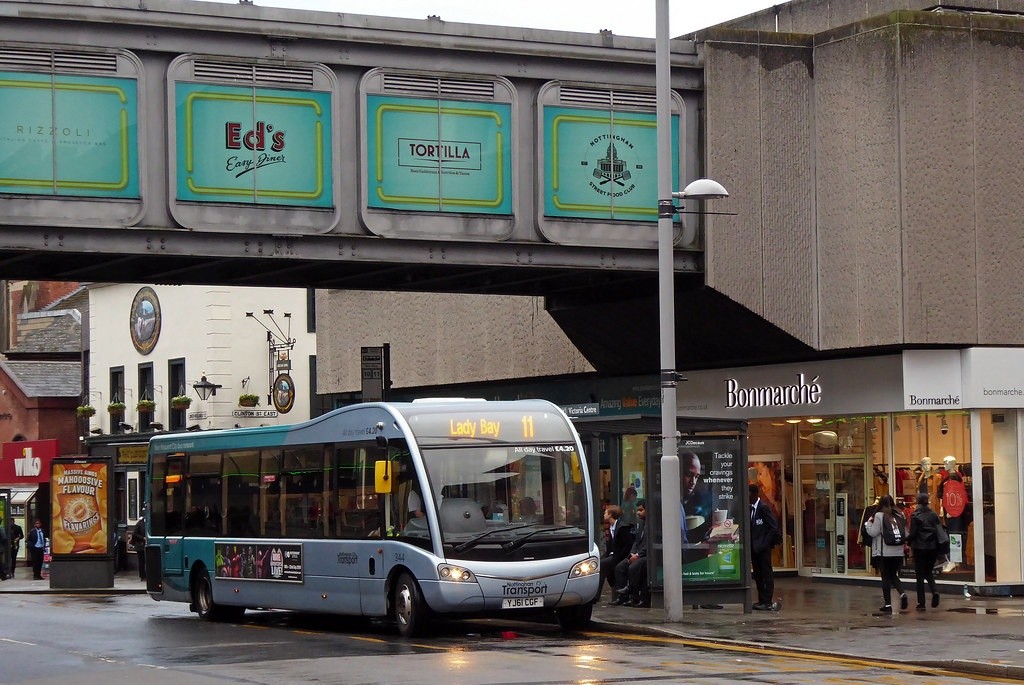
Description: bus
xmin=144 ymin=396 xmax=602 ymax=636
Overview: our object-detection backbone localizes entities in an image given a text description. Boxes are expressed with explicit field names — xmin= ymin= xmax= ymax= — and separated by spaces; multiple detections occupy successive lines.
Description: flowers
xmin=171 ymin=395 xmax=193 ymax=408
xmin=237 ymin=393 xmax=261 ymax=408
xmin=136 ymin=399 xmax=156 ymax=412
xmin=107 ymin=401 xmax=127 ymax=412
xmin=76 ymin=405 xmax=96 ymax=418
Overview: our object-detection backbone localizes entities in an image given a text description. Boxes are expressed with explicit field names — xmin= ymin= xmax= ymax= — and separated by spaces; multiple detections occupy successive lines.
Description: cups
xmin=685 ymin=515 xmax=705 ymax=529
xmin=713 ymin=510 xmax=728 ymax=525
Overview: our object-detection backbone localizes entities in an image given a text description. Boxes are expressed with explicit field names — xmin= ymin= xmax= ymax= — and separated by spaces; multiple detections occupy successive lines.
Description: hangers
xmin=851 ymin=462 xmax=915 ymax=477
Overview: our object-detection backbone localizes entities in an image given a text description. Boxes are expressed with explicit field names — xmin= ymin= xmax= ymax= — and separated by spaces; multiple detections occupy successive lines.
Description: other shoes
xmin=943 ymin=562 xmax=960 ymax=572
xmin=34 ymin=576 xmax=44 ymax=580
xmin=932 ymin=594 xmax=939 ymax=607
xmin=700 ymin=603 xmax=724 ymax=609
xmin=879 ymin=605 xmax=892 ymax=612
xmin=901 ymin=594 xmax=908 ymax=608
xmin=916 ymin=603 xmax=926 ymax=609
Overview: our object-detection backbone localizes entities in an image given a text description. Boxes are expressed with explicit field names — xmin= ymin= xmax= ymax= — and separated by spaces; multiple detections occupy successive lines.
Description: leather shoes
xmin=753 ymin=603 xmax=773 ymax=609
xmin=617 ymin=585 xmax=632 ymax=594
xmin=607 ymin=598 xmax=624 ymax=606
xmin=622 ymin=600 xmax=638 ymax=607
xmin=633 ymin=601 xmax=650 ymax=608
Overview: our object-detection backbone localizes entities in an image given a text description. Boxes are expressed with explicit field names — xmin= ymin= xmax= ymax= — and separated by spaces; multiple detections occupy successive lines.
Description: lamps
xmin=806 ymin=416 xmax=823 ymax=423
xmin=914 ymin=416 xmax=924 ymax=431
xmin=149 ymin=422 xmax=163 ymax=430
xmin=192 ymin=375 xmax=222 ymax=401
xmin=869 ymin=418 xmax=878 ymax=434
xmin=785 ymin=417 xmax=802 ymax=423
xmin=90 ymin=427 xmax=102 ymax=434
xmin=118 ymin=421 xmax=134 ymax=430
xmin=965 ymin=415 xmax=971 ymax=429
xmin=185 ymin=424 xmax=200 ymax=431
xmin=893 ymin=416 xmax=900 ymax=432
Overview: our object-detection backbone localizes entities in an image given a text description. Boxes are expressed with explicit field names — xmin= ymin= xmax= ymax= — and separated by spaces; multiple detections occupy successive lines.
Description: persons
xmin=481 ymin=504 xmax=494 ymax=520
xmin=408 ymin=463 xmax=441 ymax=518
xmin=338 ymin=466 xmax=370 ymax=489
xmin=132 ymin=512 xmax=147 ymax=580
xmin=907 ymin=494 xmax=950 ymax=611
xmin=267 ymin=474 xmax=320 ymax=493
xmin=865 ymin=496 xmax=907 ymax=612
xmin=0 ymin=517 xmax=11 ymax=580
xmin=680 ymin=450 xmax=701 ymax=544
xmin=27 ymin=519 xmax=46 ymax=580
xmin=113 ymin=518 xmax=122 ymax=574
xmin=748 ymin=484 xmax=779 ymax=611
xmin=215 ymin=546 xmax=269 ymax=579
xmin=620 ymin=487 xmax=637 ymax=522
xmin=9 ymin=518 xmax=24 ymax=578
xmin=199 ymin=483 xmax=221 ymax=530
xmin=939 ymin=455 xmax=967 ymax=566
xmin=599 ymin=500 xmax=650 ymax=608
xmin=238 ymin=477 xmax=252 ymax=500
xmin=519 ymin=497 xmax=536 ymax=523
xmin=916 ymin=456 xmax=942 ymax=519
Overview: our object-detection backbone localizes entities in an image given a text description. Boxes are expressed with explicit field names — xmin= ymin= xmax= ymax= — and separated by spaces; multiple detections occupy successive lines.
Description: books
xmin=708 ymin=524 xmax=740 ymax=544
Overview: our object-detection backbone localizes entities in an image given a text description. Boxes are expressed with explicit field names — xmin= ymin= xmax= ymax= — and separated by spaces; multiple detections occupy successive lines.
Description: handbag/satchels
xmin=935 ymin=524 xmax=950 ymax=544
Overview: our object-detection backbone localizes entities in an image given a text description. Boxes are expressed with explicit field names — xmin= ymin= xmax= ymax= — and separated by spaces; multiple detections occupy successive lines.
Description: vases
xmin=138 ymin=404 xmax=154 ymax=412
xmin=173 ymin=401 xmax=190 ymax=409
xmin=80 ymin=411 xmax=94 ymax=417
xmin=241 ymin=399 xmax=256 ymax=407
xmin=108 ymin=407 xmax=124 ymax=415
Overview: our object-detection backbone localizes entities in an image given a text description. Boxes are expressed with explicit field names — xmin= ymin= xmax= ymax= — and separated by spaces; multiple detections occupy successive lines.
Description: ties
xmin=39 ymin=531 xmax=43 ymax=544
xmin=751 ymin=507 xmax=755 ymax=521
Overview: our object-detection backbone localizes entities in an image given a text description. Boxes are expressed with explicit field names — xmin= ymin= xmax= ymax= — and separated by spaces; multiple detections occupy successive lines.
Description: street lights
xmin=659 ymin=178 xmax=731 ymax=623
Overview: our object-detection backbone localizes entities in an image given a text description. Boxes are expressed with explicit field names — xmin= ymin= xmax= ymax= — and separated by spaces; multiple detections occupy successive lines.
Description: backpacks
xmin=874 ymin=510 xmax=905 ymax=545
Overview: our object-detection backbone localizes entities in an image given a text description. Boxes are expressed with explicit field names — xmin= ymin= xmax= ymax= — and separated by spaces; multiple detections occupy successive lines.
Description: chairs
xmin=165 ymin=510 xmax=183 ymax=534
xmin=216 ymin=512 xmax=257 ymax=537
xmin=185 ymin=508 xmax=211 ymax=530
